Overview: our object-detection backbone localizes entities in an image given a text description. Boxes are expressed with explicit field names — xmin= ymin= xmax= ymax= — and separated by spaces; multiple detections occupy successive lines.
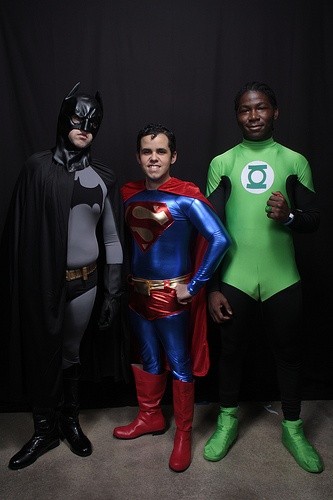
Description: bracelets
xmin=284 ymin=211 xmax=295 ymax=227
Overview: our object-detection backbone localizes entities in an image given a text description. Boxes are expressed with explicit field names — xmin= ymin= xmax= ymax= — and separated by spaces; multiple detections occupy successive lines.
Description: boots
xmin=169 ymin=380 xmax=193 ymax=472
xmin=60 ymin=377 xmax=92 ymax=457
xmin=8 ymin=377 xmax=63 ymax=470
xmin=113 ymin=365 xmax=169 ymax=439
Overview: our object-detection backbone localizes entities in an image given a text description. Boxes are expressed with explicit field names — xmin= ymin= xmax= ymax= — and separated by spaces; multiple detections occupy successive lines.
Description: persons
xmin=8 ymin=80 xmax=129 ymax=472
xmin=206 ymin=83 xmax=325 ymax=473
xmin=108 ymin=124 xmax=232 ymax=473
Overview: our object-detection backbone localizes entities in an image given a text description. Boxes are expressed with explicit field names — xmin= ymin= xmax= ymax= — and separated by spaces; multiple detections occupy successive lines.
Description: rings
xmin=265 ymin=204 xmax=273 ymax=213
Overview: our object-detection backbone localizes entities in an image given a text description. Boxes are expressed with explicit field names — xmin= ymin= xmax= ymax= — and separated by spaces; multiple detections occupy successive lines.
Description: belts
xmin=65 ymin=262 xmax=99 ymax=281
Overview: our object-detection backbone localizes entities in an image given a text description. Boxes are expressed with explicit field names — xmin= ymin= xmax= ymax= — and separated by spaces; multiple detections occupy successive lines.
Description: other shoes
xmin=203 ymin=407 xmax=240 ymax=461
xmin=281 ymin=419 xmax=324 ymax=472
